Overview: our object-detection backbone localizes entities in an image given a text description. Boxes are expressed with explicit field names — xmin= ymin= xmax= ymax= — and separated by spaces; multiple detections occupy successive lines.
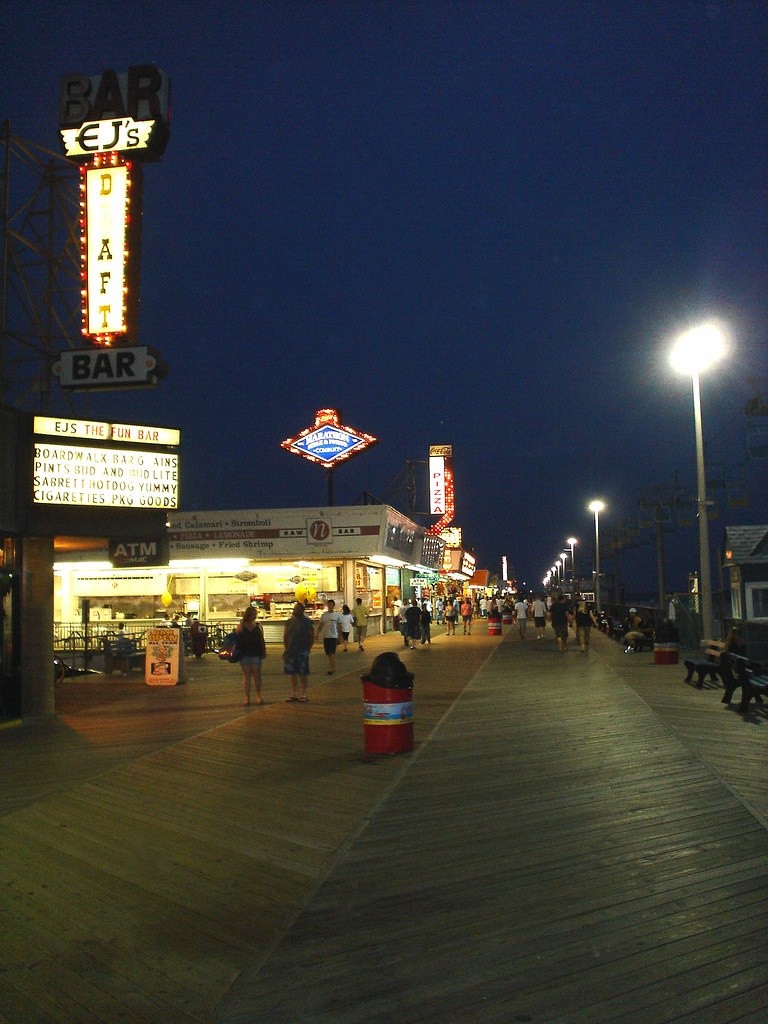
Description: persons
xmin=352 ymin=598 xmax=370 ymax=652
xmin=316 ymin=599 xmax=343 ymax=675
xmin=399 ymin=598 xmax=432 ymax=650
xmin=622 ymin=607 xmax=655 ymax=654
xmin=340 ymin=605 xmax=354 ymax=651
xmin=527 ymin=595 xmax=555 ymax=612
xmin=569 ymin=591 xmax=599 ymax=652
xmin=472 ymin=593 xmax=504 ymax=619
xmin=723 ymin=626 xmax=746 ymax=657
xmin=159 ymin=612 xmax=193 ymax=626
xmin=284 ymin=603 xmax=316 ymax=703
xmin=503 ymin=594 xmax=529 ymax=639
xmin=435 ymin=594 xmax=472 ymax=635
xmin=234 ymin=607 xmax=265 ymax=705
xmin=550 ymin=595 xmax=573 ymax=652
xmin=531 ymin=596 xmax=548 ymax=640
xmin=390 ymin=596 xmax=403 ymax=630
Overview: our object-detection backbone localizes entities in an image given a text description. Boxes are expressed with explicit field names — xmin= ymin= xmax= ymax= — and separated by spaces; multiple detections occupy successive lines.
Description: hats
xmin=629 ymin=608 xmax=637 ymax=613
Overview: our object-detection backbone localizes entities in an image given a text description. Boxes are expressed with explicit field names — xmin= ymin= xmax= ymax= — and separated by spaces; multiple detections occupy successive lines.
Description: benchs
xmin=685 ymin=638 xmax=726 ymax=689
xmin=718 ymin=649 xmax=768 ymax=712
xmin=636 ymin=629 xmax=655 ymax=652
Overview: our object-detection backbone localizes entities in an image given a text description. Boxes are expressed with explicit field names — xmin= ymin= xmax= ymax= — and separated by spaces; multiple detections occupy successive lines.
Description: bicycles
xmin=208 ymin=620 xmax=230 ymax=654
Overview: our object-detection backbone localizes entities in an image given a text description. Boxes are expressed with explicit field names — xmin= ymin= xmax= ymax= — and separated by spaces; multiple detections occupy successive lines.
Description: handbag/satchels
xmin=219 ymin=639 xmax=245 ymax=663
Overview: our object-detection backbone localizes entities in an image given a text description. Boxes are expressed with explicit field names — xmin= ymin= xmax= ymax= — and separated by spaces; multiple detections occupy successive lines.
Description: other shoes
xmin=285 ymin=696 xmax=297 ymax=702
xmin=256 ymin=699 xmax=263 ymax=704
xmin=243 ymin=700 xmax=250 ymax=706
xmin=468 ymin=632 xmax=470 ymax=635
xmin=298 ymin=696 xmax=308 ymax=701
xmin=541 ymin=635 xmax=543 ymax=638
xmin=537 ymin=636 xmax=540 ymax=639
xmin=624 ymin=646 xmax=634 ymax=653
xmin=463 ymin=630 xmax=466 ymax=634
xmin=360 ymin=645 xmax=364 ymax=651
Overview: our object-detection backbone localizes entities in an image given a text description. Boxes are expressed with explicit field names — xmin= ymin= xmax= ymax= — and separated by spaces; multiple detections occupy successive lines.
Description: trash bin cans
xmin=361 ymin=651 xmax=414 ymax=754
xmin=488 ymin=610 xmax=502 ymax=636
xmin=502 ymin=606 xmax=512 ymax=625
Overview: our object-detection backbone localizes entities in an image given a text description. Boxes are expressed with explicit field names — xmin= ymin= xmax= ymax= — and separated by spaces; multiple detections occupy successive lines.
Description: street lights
xmin=588 ymin=500 xmax=603 ymax=612
xmin=551 ymin=566 xmax=557 ymax=578
xmin=560 ymin=553 xmax=567 ymax=580
xmin=567 ymin=537 xmax=576 ymax=591
xmin=665 ymin=325 xmax=715 ymax=657
xmin=555 ymin=560 xmax=561 ymax=585
